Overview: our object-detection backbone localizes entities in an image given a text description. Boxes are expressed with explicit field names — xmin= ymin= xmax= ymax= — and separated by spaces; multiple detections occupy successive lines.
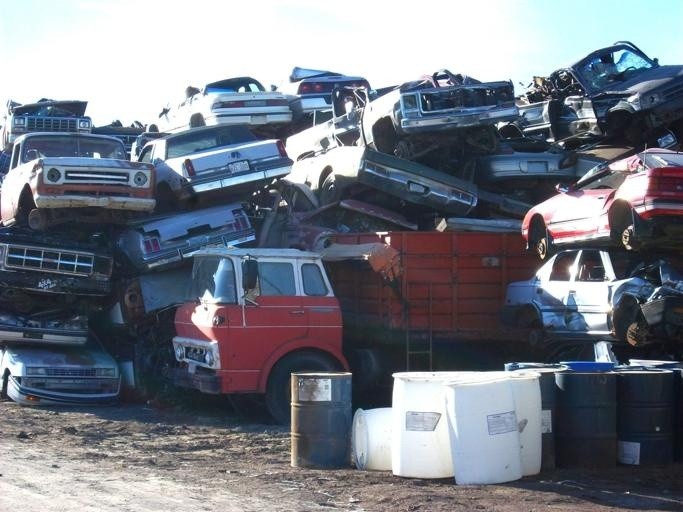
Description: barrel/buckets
xmin=354 ymin=359 xmax=683 ymax=485
xmin=290 ymin=373 xmax=353 ymax=470
